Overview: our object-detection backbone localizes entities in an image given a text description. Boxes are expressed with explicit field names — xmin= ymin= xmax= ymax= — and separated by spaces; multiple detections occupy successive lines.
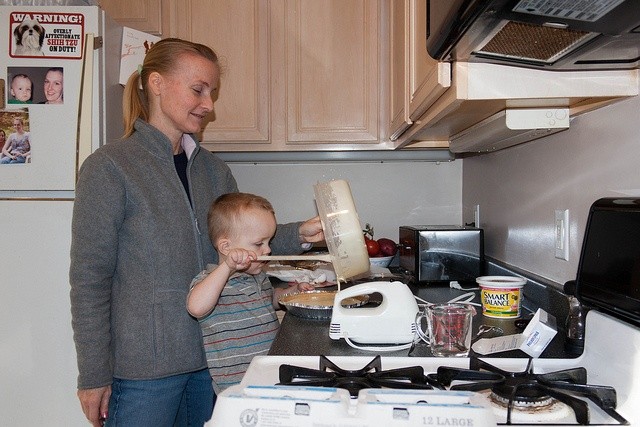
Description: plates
xmin=277 ymin=290 xmax=370 ymax=320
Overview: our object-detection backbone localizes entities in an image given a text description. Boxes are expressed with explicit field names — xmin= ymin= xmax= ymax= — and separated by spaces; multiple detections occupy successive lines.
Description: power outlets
xmin=554 ymin=209 xmax=570 ymax=261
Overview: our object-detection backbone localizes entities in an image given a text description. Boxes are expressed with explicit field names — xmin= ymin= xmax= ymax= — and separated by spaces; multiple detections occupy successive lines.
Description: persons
xmin=7 ymin=73 xmax=34 ymax=104
xmin=0 ymin=117 xmax=31 ymax=164
xmin=0 ymin=130 xmax=5 ymax=153
xmin=37 ymin=67 xmax=63 ymax=104
xmin=183 ymin=191 xmax=318 ymax=397
xmin=68 ymin=36 xmax=325 ymax=427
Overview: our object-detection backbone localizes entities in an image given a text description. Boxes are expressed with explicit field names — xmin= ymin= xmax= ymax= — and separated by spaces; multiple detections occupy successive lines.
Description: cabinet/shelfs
xmin=393 ymin=0 xmax=640 ymax=150
xmin=99 ymin=0 xmax=166 ymax=38
xmin=166 ymin=0 xmax=393 ymax=152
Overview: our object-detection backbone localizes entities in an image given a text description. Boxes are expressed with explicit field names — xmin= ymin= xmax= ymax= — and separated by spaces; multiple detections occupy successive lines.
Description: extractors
xmin=425 ymin=0 xmax=640 ymax=71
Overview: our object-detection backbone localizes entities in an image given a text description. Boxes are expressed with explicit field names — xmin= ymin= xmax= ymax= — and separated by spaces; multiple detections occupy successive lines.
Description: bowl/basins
xmin=312 ymin=179 xmax=370 ymax=281
xmin=369 ymin=256 xmax=394 ymax=268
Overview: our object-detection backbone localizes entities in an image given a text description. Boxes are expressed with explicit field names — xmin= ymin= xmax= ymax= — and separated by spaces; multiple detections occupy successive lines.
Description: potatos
xmin=377 ymin=238 xmax=397 ymax=255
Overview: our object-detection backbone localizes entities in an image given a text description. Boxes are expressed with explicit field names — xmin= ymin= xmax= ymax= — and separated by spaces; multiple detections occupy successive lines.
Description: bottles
xmin=564 ymin=297 xmax=586 ymax=356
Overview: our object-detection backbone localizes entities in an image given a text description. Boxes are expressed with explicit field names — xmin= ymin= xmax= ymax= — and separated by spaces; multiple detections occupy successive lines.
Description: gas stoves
xmin=205 ymin=310 xmax=640 ymax=427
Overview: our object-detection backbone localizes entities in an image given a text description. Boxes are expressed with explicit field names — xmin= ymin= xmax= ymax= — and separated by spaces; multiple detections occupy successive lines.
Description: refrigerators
xmin=0 ymin=5 xmax=147 ymax=427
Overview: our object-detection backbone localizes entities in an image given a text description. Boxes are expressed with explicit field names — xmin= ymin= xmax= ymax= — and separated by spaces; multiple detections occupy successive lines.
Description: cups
xmin=414 ymin=303 xmax=473 ymax=358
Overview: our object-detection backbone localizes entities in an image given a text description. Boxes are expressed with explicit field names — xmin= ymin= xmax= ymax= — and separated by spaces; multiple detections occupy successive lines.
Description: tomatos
xmin=363 ymin=224 xmax=379 ymax=256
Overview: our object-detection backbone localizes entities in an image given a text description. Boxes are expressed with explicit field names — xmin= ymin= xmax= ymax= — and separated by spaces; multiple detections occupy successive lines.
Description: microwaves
xmin=399 ymin=224 xmax=485 ymax=285
